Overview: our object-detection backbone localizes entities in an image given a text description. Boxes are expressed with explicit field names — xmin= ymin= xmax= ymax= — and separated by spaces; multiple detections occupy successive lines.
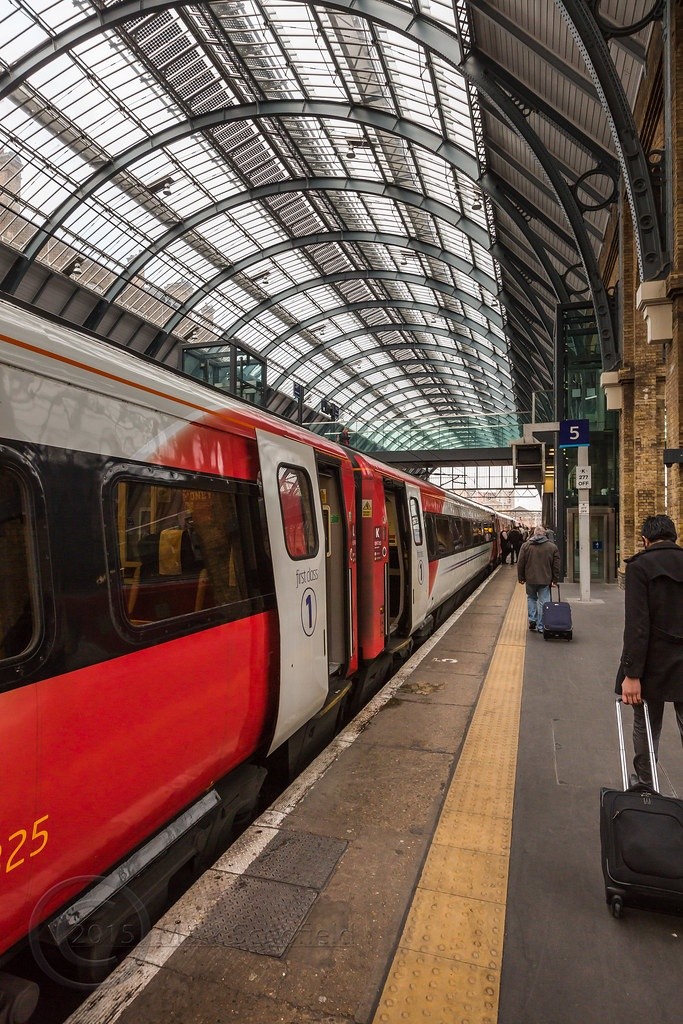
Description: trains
xmin=1 ymin=297 xmax=518 ymax=949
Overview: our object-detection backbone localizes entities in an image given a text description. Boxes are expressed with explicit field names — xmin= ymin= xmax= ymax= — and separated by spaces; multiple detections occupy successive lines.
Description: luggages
xmin=600 ymin=695 xmax=683 ymax=923
xmin=542 ymin=582 xmax=574 ymax=641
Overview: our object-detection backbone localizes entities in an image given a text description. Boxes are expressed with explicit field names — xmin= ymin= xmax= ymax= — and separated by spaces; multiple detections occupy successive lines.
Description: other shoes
xmin=502 ymin=562 xmax=509 ymax=565
xmin=510 ymin=562 xmax=514 ymax=565
xmin=529 ymin=621 xmax=537 ymax=631
xmin=628 ymin=772 xmax=639 ymax=787
xmin=537 ymin=629 xmax=543 ymax=633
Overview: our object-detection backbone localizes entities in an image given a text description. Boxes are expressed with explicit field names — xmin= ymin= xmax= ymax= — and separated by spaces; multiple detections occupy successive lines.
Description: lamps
xmin=72 ymin=144 xmax=500 ymax=339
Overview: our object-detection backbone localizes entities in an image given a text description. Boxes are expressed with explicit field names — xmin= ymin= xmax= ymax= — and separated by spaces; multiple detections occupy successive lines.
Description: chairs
xmin=122 ymin=528 xmax=210 ymax=627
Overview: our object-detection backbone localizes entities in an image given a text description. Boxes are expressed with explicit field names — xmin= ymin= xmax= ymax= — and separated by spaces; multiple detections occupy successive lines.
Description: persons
xmin=614 ymin=514 xmax=683 ymax=790
xmin=501 ymin=524 xmax=554 ymax=565
xmin=517 ymin=526 xmax=560 ymax=632
